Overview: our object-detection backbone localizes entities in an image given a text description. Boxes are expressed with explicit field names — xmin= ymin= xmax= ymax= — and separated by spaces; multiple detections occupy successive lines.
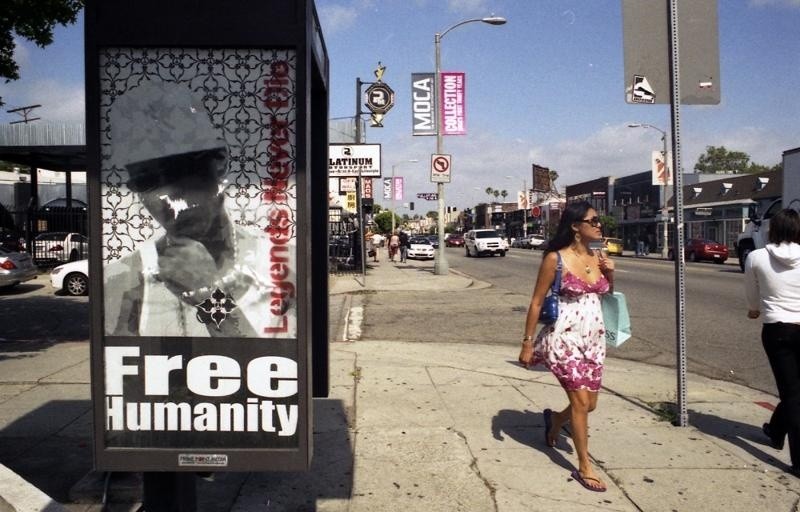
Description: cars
xmin=327 ymin=233 xmax=375 ymax=258
xmin=666 ymin=239 xmax=729 ymax=265
xmin=51 ymin=256 xmax=90 ymax=296
xmin=22 ymin=227 xmax=89 ymax=265
xmin=387 ymin=221 xmax=623 ymax=262
xmin=0 ymin=247 xmax=39 ymax=290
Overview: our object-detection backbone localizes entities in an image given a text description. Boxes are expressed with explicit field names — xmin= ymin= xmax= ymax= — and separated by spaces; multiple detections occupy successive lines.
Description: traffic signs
xmin=416 ymin=191 xmax=438 ymax=200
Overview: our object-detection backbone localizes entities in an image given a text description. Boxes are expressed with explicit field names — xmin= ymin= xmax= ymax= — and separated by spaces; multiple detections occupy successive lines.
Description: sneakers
xmin=763 ymin=423 xmax=783 ymax=449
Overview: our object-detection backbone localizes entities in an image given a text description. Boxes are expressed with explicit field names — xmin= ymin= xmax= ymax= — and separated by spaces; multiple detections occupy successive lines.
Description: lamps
xmin=82 ymin=205 xmax=88 ymax=211
xmin=45 ymin=204 xmax=51 ymax=211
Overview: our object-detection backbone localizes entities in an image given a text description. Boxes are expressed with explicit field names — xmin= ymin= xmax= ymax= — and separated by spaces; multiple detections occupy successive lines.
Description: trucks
xmin=732 ymin=143 xmax=800 ymax=273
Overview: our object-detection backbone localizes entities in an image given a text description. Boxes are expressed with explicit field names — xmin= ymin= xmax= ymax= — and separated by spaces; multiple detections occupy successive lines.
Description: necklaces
xmin=163 ymin=221 xmax=242 ymax=305
xmin=569 ymin=246 xmax=596 ymax=274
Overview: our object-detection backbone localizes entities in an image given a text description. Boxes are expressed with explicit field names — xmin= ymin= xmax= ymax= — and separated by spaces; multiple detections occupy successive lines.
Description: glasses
xmin=574 ymin=215 xmax=604 ymax=228
xmin=124 ymin=156 xmax=202 ymax=195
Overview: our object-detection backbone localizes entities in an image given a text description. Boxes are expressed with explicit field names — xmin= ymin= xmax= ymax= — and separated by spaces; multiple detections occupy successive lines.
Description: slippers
xmin=572 ymin=468 xmax=606 ymax=493
xmin=544 ymin=409 xmax=561 ymax=447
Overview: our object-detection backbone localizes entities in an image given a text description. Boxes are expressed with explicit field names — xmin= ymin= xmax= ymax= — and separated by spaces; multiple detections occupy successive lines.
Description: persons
xmin=742 ymin=204 xmax=799 ymax=479
xmin=388 ymin=234 xmax=399 ymax=263
xmin=100 ymin=81 xmax=300 ymax=341
xmin=517 ymin=198 xmax=616 ymax=494
xmin=367 ymin=235 xmax=379 ymax=263
xmin=399 ymin=230 xmax=409 ymax=263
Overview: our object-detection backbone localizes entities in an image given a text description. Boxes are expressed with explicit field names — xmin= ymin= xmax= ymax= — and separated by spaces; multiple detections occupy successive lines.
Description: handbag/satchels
xmin=600 ymin=292 xmax=631 ymax=347
xmin=538 ymin=293 xmax=557 ymax=322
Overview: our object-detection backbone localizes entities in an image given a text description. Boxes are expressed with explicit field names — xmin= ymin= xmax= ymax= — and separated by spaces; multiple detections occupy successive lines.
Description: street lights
xmin=505 ymin=174 xmax=527 ymax=239
xmin=629 ymin=123 xmax=668 ymax=259
xmin=391 ymin=158 xmax=420 ymax=237
xmin=432 ymin=15 xmax=508 ymax=275
xmin=471 ymin=185 xmax=492 ymax=229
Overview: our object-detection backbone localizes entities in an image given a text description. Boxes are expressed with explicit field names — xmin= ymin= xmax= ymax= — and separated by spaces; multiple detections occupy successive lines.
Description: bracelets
xmin=192 ymin=287 xmax=239 ymax=328
xmin=523 ymin=334 xmax=532 ymax=342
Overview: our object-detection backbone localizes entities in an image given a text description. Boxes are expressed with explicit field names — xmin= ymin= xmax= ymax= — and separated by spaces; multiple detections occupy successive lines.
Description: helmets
xmin=110 ymin=81 xmax=228 ymax=168
